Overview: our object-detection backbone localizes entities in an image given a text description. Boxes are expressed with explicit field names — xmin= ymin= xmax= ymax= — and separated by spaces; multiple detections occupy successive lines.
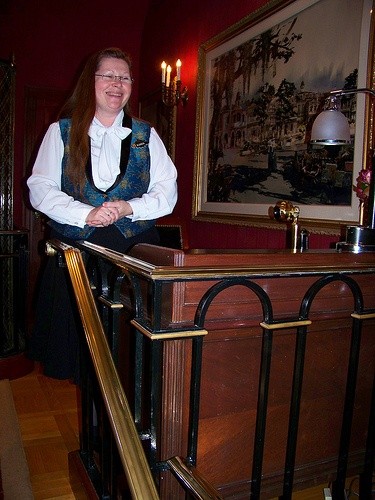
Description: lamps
xmin=310 ymin=88 xmax=375 ymax=254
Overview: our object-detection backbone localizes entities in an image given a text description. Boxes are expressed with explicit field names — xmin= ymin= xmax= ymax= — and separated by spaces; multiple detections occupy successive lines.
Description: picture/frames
xmin=137 ymin=76 xmax=177 ymax=163
xmin=191 ymin=1 xmax=375 ymax=237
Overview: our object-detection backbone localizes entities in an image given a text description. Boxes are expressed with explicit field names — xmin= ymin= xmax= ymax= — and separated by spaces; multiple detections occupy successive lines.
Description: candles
xmin=176 ymin=59 xmax=182 ymax=80
xmin=161 ymin=60 xmax=167 ymax=83
xmin=166 ymin=65 xmax=172 ymax=87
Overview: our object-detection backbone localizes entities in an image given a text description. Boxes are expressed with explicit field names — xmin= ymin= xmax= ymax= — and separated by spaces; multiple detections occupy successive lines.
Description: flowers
xmin=352 ymin=169 xmax=372 ymax=205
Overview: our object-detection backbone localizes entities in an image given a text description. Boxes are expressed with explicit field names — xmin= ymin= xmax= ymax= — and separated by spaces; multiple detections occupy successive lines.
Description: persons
xmin=26 ymin=46 xmax=178 ymax=379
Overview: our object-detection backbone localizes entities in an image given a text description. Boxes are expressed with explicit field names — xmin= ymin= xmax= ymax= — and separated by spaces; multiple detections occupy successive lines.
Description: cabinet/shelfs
xmin=117 ymin=244 xmax=375 ymax=500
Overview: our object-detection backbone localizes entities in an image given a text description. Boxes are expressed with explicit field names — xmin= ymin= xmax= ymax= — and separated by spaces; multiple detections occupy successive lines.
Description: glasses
xmin=95 ymin=72 xmax=134 ymax=84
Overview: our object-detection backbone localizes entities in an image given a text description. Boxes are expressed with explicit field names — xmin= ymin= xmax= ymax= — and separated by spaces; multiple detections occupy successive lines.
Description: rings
xmin=107 ymin=211 xmax=112 ymax=216
